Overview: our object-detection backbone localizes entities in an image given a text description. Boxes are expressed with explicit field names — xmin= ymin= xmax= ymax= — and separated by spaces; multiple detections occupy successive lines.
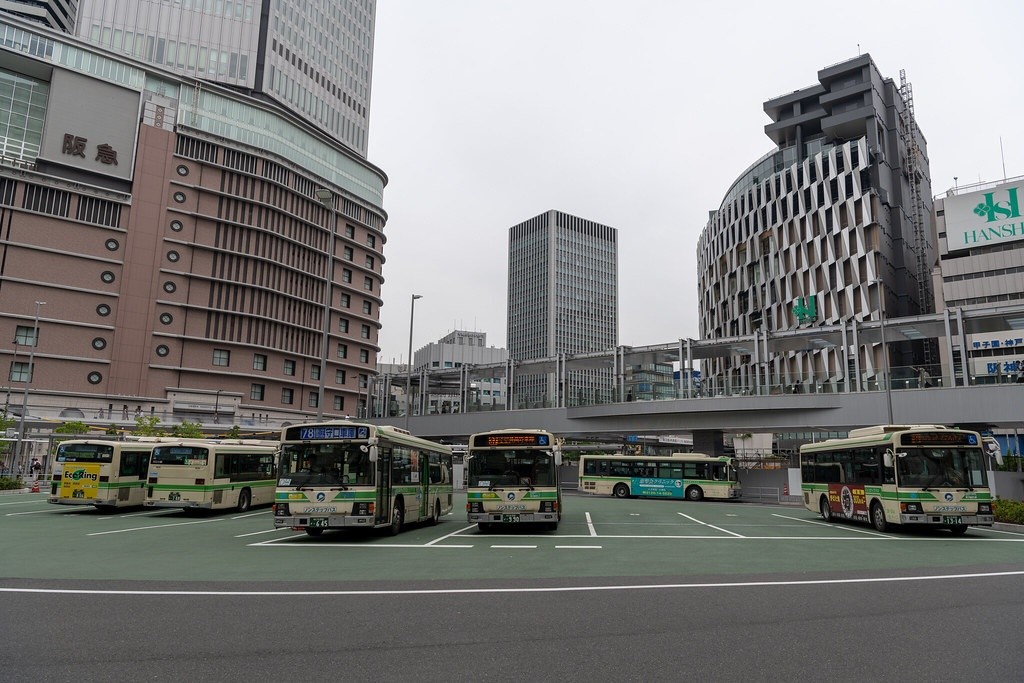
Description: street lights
xmin=871 ymin=277 xmax=893 ymax=426
xmin=12 ymin=301 xmax=47 ymax=482
xmin=214 ymin=389 xmax=224 ymax=423
xmin=315 ymin=188 xmax=336 ymax=423
xmin=405 ymin=294 xmax=424 ymax=429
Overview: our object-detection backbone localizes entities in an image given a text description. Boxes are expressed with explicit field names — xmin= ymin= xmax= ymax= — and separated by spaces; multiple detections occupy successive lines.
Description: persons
xmin=433 ymin=402 xmax=446 ymax=414
xmin=1016 ymin=360 xmax=1024 ymax=383
xmin=795 ymin=380 xmax=799 ymax=394
xmin=32 ymin=459 xmax=42 ymax=482
xmin=301 ymin=453 xmax=326 ymax=473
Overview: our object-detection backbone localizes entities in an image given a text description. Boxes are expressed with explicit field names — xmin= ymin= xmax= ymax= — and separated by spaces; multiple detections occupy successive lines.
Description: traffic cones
xmin=29 ymin=479 xmax=41 ymax=493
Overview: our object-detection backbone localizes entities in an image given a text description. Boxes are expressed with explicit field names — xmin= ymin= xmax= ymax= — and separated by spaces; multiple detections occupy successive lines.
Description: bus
xmin=46 ymin=439 xmax=157 ymax=512
xmin=463 ymin=428 xmax=563 ymax=531
xmin=391 ymin=459 xmax=449 ymax=486
xmin=799 ymin=423 xmax=1005 ymax=533
xmin=579 ymin=452 xmax=749 ymax=502
xmin=143 ymin=441 xmax=309 ymax=513
xmin=267 ymin=420 xmax=453 ymax=535
